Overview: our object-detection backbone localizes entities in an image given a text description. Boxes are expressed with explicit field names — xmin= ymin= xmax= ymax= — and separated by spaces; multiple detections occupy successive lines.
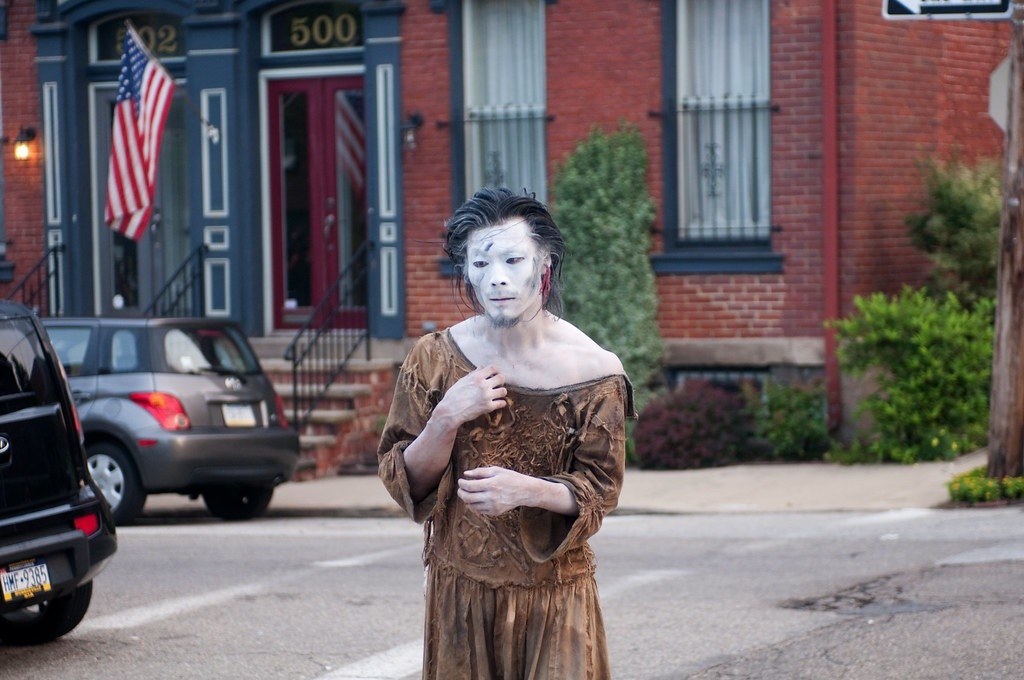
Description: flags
xmin=103 ymin=28 xmax=172 ymax=239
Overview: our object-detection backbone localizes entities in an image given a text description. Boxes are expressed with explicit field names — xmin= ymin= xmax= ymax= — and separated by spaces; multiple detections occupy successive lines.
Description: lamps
xmin=14 ymin=126 xmax=36 ymax=159
xmin=401 ymin=112 xmax=425 ymax=152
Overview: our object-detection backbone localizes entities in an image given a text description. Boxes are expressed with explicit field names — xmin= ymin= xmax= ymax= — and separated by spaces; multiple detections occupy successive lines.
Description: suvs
xmin=36 ymin=314 xmax=299 ymax=528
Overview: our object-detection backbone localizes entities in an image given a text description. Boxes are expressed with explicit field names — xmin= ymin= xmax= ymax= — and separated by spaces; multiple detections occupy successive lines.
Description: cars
xmin=0 ymin=299 xmax=119 ymax=649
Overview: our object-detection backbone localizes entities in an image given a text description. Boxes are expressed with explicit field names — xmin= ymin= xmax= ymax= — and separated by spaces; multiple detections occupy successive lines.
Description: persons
xmin=377 ymin=186 xmax=636 ymax=680
